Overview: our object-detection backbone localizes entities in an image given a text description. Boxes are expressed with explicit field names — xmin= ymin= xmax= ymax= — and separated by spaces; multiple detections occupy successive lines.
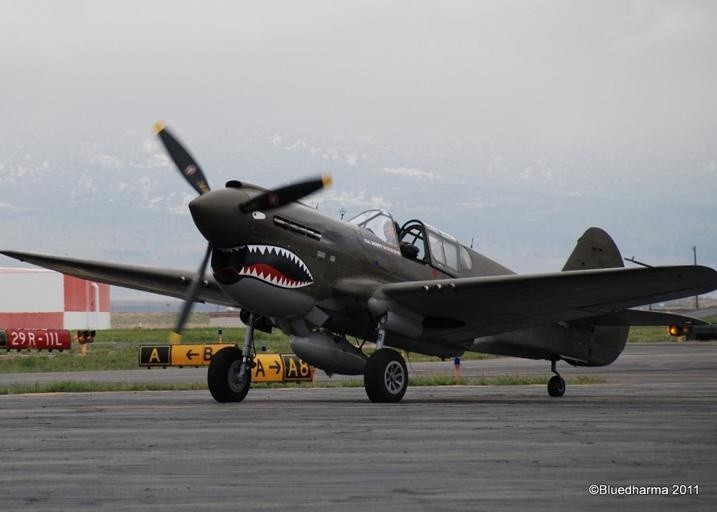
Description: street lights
xmin=689 ymin=245 xmax=698 ymax=309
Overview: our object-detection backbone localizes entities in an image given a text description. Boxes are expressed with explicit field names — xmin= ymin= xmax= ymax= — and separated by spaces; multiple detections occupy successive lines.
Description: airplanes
xmin=0 ymin=122 xmax=717 ymax=403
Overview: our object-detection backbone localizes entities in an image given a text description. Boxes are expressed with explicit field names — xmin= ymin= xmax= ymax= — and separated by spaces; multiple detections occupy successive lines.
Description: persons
xmin=384 ymin=221 xmax=419 ymax=259
